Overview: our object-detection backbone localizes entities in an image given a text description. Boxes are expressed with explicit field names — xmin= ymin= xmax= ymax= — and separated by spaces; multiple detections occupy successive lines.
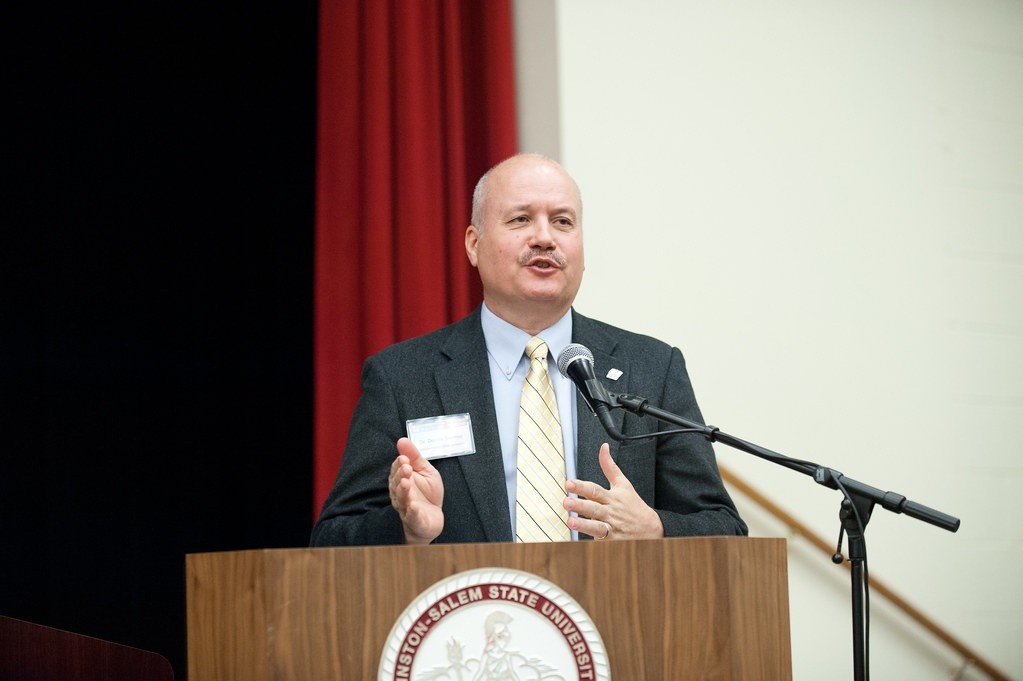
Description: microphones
xmin=556 ymin=343 xmax=625 ymax=441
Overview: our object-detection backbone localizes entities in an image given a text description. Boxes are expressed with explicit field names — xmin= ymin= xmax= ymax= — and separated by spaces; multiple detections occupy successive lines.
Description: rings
xmin=598 ymin=521 xmax=609 ymax=540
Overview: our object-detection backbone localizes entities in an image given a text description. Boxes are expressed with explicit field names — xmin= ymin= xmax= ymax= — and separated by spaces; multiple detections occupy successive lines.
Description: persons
xmin=308 ymin=153 xmax=746 ymax=551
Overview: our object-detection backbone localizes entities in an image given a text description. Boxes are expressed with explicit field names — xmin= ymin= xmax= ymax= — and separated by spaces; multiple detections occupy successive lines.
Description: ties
xmin=515 ymin=336 xmax=572 ymax=543
